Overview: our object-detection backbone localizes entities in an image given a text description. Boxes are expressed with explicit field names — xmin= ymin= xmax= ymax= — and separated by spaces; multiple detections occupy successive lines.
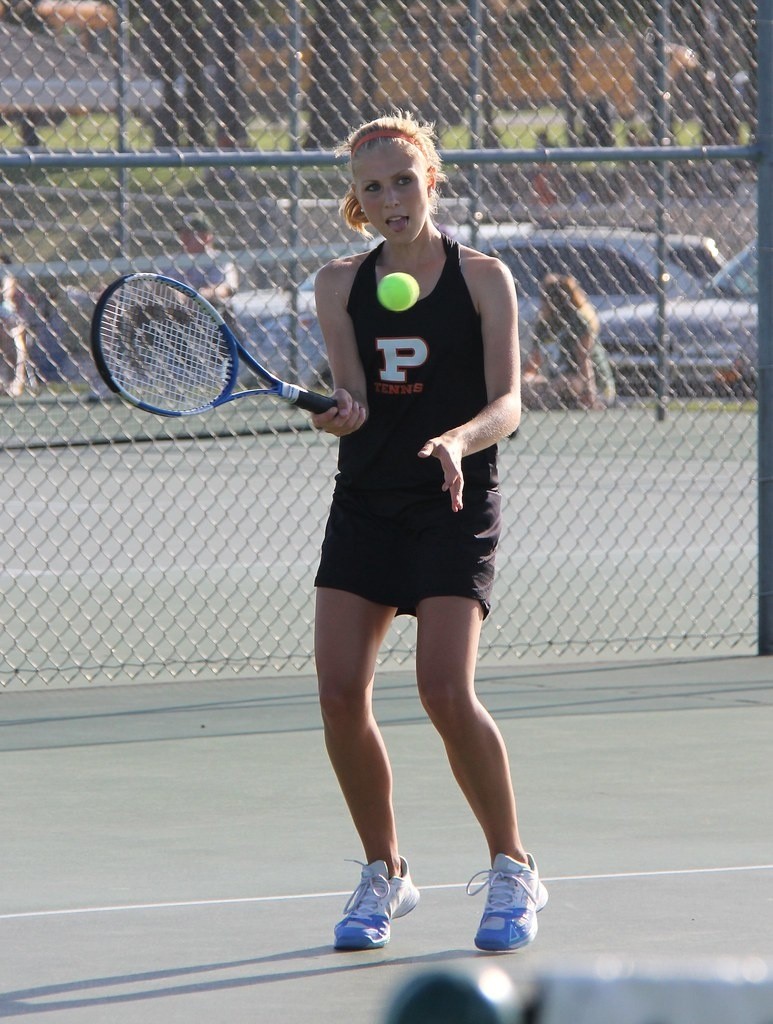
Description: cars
xmin=208 ymin=219 xmax=762 ymax=400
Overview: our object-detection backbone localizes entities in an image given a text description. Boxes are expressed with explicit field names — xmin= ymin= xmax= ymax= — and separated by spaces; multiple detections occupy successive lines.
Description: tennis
xmin=376 ymin=271 xmax=421 ymax=313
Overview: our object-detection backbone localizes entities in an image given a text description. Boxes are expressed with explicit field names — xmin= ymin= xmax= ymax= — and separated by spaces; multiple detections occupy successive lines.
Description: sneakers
xmin=464 ymin=853 xmax=549 ymax=952
xmin=333 ymin=855 xmax=421 ymax=951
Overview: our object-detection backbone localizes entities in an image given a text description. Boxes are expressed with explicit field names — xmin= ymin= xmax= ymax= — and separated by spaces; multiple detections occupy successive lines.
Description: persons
xmin=312 ymin=115 xmax=549 ymax=951
xmin=118 ymin=212 xmax=238 ymax=389
xmin=531 ymin=98 xmax=656 ymax=214
xmin=0 ymin=274 xmax=30 ymax=398
xmin=525 ymin=272 xmax=615 ymax=411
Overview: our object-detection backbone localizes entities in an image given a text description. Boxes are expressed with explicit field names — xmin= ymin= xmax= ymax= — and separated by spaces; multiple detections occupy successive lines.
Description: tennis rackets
xmin=90 ymin=270 xmax=363 ymax=425
